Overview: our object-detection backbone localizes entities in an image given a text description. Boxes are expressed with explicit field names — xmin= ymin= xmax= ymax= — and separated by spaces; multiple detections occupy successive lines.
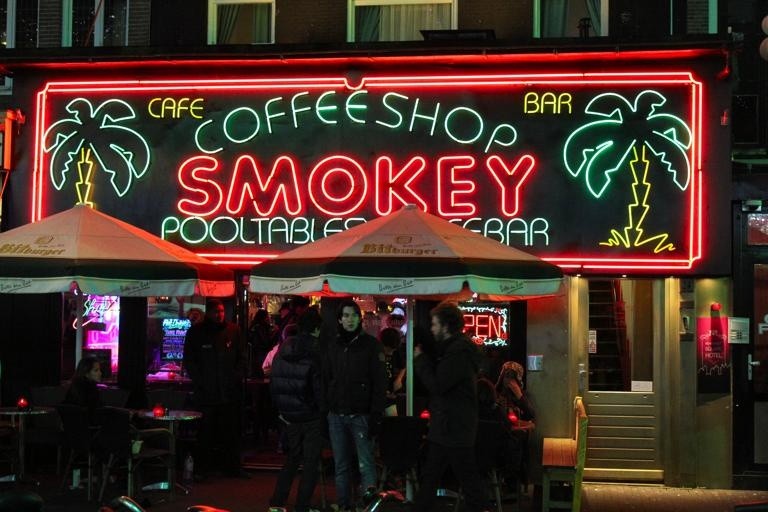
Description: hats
xmin=501 ymin=360 xmax=525 ymax=381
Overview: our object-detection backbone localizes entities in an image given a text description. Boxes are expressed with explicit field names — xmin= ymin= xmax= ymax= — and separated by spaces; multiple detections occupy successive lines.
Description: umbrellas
xmin=248 ymin=203 xmax=564 ymax=417
xmin=0 ymin=202 xmax=235 ymax=369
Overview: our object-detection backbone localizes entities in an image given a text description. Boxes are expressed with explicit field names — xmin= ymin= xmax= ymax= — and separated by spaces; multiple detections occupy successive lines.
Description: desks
xmin=0 ymin=406 xmax=56 ymax=484
xmin=138 ymin=407 xmax=204 ymax=499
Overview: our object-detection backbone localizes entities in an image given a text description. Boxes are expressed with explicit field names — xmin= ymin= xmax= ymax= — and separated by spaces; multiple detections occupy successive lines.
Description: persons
xmin=55 ymin=356 xmax=133 ymax=494
xmin=182 ymin=296 xmax=537 ymax=512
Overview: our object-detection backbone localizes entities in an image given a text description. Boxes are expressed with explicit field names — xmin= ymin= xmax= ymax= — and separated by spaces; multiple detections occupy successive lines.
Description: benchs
xmin=540 ymin=396 xmax=590 ymax=512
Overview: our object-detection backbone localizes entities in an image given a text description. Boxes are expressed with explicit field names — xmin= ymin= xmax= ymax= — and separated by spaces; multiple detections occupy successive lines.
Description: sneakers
xmin=309 ymin=508 xmax=319 ymax=512
xmin=269 ymin=507 xmax=288 ymax=511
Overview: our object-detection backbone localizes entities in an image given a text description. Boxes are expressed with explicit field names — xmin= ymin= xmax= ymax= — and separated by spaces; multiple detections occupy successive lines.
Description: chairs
xmin=96 ymin=403 xmax=147 ymax=500
xmin=57 ymin=402 xmax=97 ymax=503
